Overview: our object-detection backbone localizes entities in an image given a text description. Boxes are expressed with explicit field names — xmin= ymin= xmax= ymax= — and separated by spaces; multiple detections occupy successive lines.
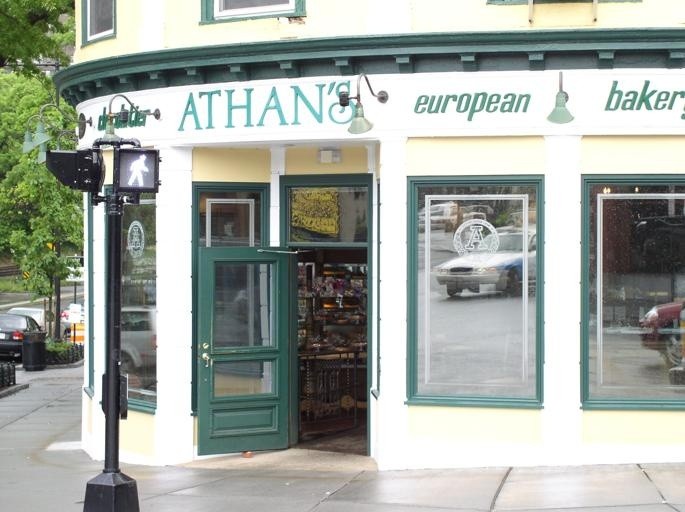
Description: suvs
xmin=630 ymin=216 xmax=684 ymax=273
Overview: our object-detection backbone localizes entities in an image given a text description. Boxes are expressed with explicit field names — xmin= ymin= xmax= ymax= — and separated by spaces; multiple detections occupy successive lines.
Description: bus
xmin=65 ymin=256 xmax=84 ymax=282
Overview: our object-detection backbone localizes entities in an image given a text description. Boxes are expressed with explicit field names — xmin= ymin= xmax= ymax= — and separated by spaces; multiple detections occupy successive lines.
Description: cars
xmin=417 ymin=203 xmax=486 ymax=231
xmin=436 ymin=233 xmax=536 ymax=296
xmin=0 ymin=306 xmax=84 ymax=363
xmin=119 ymin=306 xmax=156 ymax=370
xmin=641 ymin=304 xmax=685 ymax=366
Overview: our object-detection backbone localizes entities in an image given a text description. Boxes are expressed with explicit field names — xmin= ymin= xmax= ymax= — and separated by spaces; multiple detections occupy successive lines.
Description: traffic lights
xmin=111 ymin=147 xmax=158 ymax=193
xmin=44 ymin=149 xmax=93 ymax=192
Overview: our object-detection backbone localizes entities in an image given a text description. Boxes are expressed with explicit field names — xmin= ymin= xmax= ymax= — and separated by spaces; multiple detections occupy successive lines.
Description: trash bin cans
xmin=23 ymin=331 xmax=47 ymax=371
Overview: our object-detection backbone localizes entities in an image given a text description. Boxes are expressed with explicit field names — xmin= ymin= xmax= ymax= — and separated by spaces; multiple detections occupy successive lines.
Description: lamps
xmin=542 ymin=71 xmax=577 ymax=127
xmin=97 ymin=94 xmax=161 ymax=146
xmin=21 ymin=103 xmax=93 ymax=167
xmin=338 ymin=71 xmax=388 ymax=136
